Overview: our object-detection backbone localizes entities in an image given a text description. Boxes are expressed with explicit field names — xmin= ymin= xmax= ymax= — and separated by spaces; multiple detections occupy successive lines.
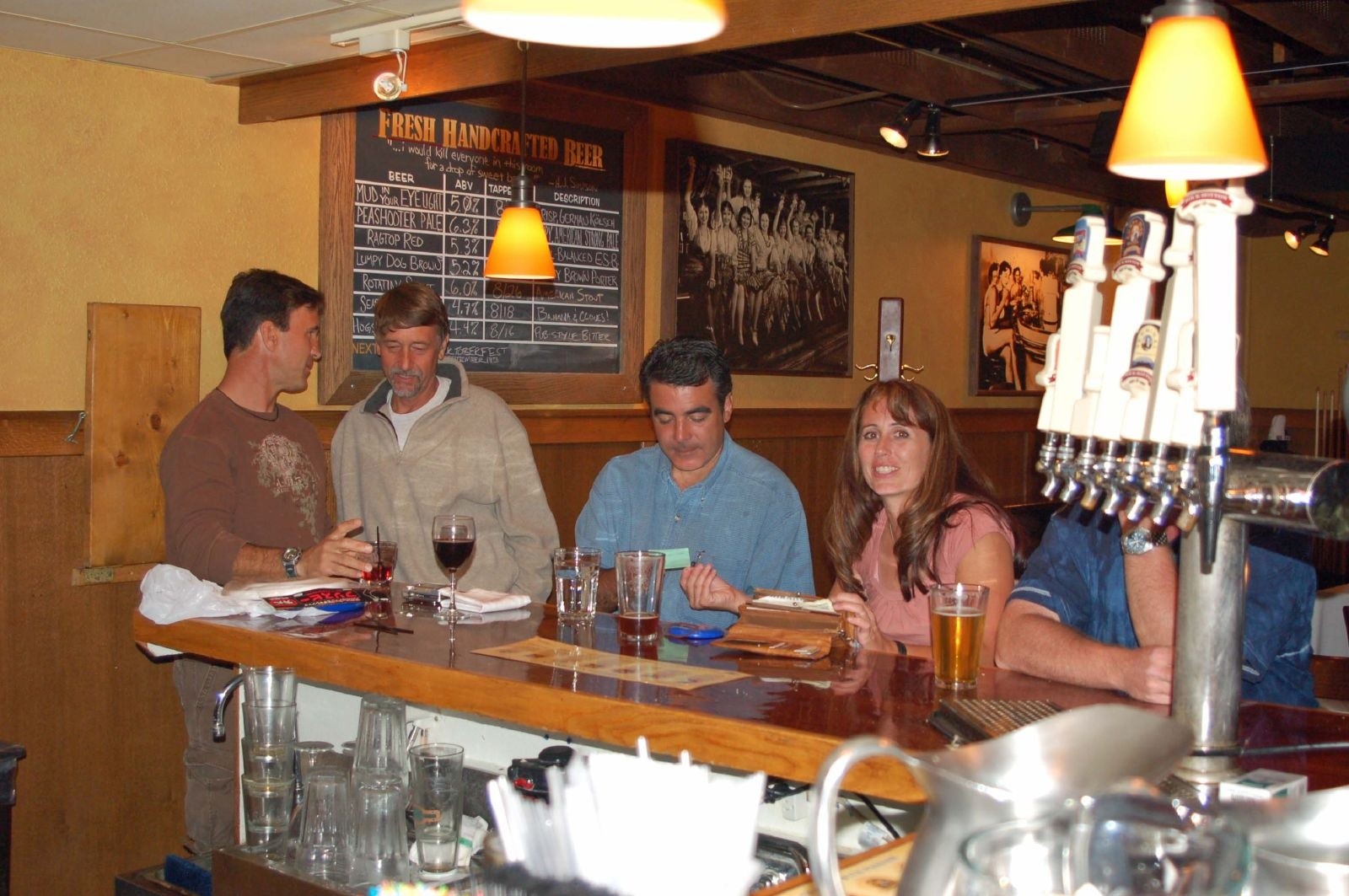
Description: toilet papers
xmin=1268 ymin=414 xmax=1287 ymax=440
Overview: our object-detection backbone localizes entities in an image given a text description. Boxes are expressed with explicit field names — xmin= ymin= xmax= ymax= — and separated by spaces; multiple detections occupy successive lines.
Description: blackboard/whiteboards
xmin=354 ymin=102 xmax=623 ymax=377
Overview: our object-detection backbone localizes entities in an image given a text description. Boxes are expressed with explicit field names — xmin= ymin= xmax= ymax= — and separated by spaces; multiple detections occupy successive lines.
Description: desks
xmin=137 ymin=574 xmax=1176 ymax=896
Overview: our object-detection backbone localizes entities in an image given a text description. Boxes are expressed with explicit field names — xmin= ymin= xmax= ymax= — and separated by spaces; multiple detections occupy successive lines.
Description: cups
xmin=241 ymin=666 xmax=464 ymax=880
xmin=356 ymin=540 xmax=397 ymax=585
xmin=553 ymin=547 xmax=600 ymax=621
xmin=615 ymin=551 xmax=665 ymax=643
xmin=929 ymin=583 xmax=990 ymax=690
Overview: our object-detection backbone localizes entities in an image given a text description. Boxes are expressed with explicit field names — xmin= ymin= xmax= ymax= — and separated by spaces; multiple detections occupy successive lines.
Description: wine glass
xmin=431 ymin=514 xmax=476 ymax=619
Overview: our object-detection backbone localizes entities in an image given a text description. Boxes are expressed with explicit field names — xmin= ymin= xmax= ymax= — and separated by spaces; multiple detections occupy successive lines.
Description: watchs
xmin=1121 ymin=526 xmax=1168 ymax=555
xmin=281 ymin=545 xmax=302 ymax=578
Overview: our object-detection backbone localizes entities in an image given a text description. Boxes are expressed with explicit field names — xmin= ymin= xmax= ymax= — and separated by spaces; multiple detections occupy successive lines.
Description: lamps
xmin=463 ymin=0 xmax=728 ymax=50
xmin=1012 ymin=190 xmax=1123 ymax=245
xmin=1284 ymin=214 xmax=1336 ymax=256
xmin=484 ymin=40 xmax=558 ymax=280
xmin=1107 ymin=0 xmax=1269 ymax=181
xmin=358 ymin=29 xmax=411 ymax=100
xmin=879 ymin=99 xmax=950 ymax=157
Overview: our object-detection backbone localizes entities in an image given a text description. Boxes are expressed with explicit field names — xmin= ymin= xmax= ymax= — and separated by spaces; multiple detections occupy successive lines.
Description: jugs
xmin=810 ymin=706 xmax=1195 ymax=895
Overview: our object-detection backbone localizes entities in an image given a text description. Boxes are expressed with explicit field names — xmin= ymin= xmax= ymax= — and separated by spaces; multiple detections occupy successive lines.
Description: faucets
xmin=212 ymin=673 xmax=244 ymax=738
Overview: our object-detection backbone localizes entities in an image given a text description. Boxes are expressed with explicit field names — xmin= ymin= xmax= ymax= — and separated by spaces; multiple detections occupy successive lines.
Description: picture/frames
xmin=970 ymin=235 xmax=1071 ymax=396
xmin=662 ymin=138 xmax=855 ymax=378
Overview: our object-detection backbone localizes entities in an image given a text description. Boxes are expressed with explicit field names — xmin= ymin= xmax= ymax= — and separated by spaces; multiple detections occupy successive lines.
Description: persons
xmin=681 ymin=378 xmax=1022 ymax=667
xmin=158 ymin=266 xmax=373 ymax=855
xmin=330 ymin=282 xmax=560 ymax=607
xmin=680 ymin=156 xmax=850 ymax=351
xmin=575 ymin=335 xmax=817 ymax=633
xmin=995 ymin=367 xmax=1320 ymax=709
